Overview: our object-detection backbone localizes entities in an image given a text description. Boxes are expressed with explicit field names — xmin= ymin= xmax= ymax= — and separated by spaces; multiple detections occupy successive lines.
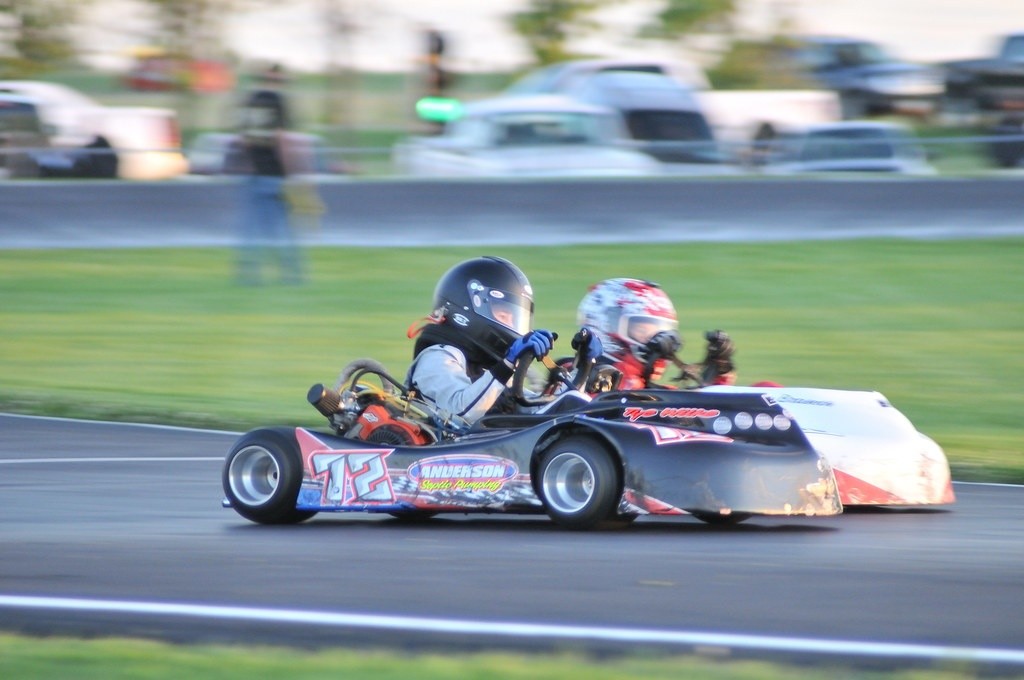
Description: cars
xmin=1 ymin=80 xmax=191 ymax=180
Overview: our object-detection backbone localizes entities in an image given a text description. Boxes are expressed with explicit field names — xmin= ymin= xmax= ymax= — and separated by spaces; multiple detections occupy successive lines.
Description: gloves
xmin=707 ymin=330 xmax=735 ymax=360
xmin=646 ymin=330 xmax=680 ymax=359
xmin=571 ymin=326 xmax=603 ymax=359
xmin=505 ymin=328 xmax=559 ymax=362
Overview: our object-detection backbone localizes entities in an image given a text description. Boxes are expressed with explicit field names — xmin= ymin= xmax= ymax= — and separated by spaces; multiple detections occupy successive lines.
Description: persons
xmin=401 ymin=255 xmax=604 ymax=436
xmin=542 ymin=276 xmax=740 ymax=398
xmin=410 ymin=29 xmax=452 ymax=138
xmin=216 ymin=48 xmax=322 ymax=294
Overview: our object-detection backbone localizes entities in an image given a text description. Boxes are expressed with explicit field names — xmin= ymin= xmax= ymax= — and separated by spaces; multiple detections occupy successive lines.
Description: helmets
xmin=433 ymin=256 xmax=534 ymax=364
xmin=576 ymin=278 xmax=679 ymax=380
